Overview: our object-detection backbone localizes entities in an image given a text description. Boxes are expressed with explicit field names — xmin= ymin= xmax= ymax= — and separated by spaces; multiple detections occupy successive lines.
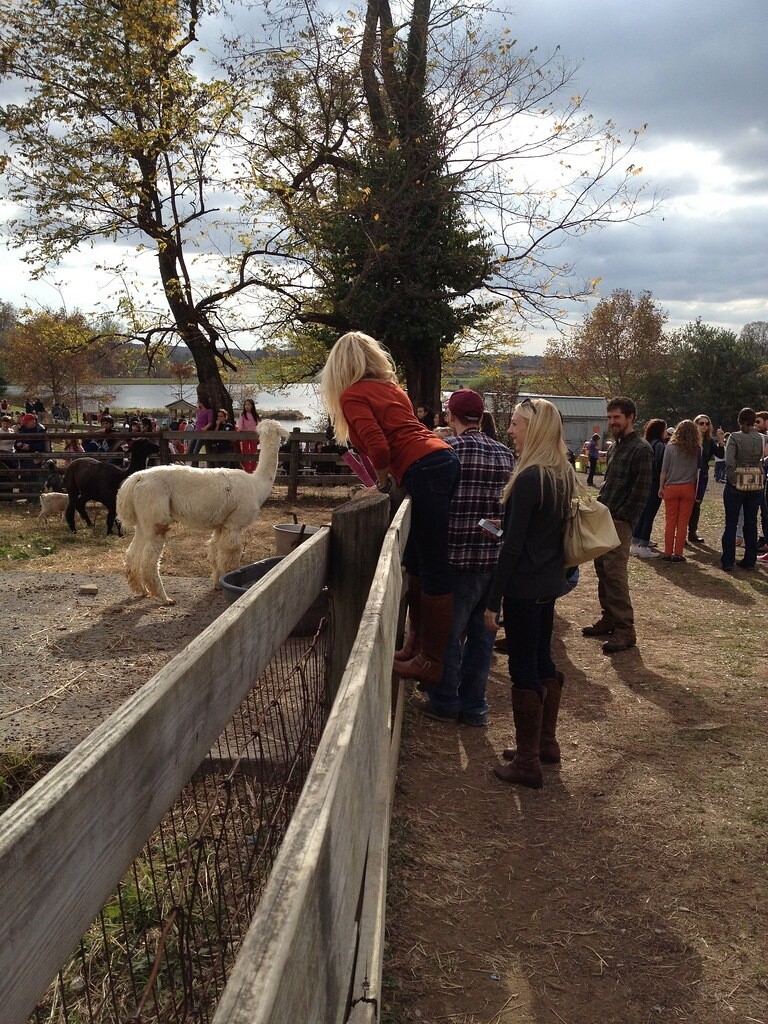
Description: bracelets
xmin=718 ymin=441 xmax=723 ymax=444
xmin=376 ymin=478 xmax=392 ymax=493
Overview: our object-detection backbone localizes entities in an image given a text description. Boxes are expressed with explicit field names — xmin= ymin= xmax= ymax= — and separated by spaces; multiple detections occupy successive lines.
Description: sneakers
xmin=629 ymin=545 xmax=660 ymax=557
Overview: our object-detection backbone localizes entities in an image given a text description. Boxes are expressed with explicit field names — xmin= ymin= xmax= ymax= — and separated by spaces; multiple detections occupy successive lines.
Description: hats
xmin=20 ymin=413 xmax=36 ymax=424
xmin=448 ymin=389 xmax=484 ymax=420
xmin=667 ymin=427 xmax=675 ymax=435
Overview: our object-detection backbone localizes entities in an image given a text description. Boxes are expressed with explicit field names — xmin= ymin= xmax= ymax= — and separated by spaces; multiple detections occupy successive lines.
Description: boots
xmin=394 ymin=573 xmax=425 ymax=662
xmin=503 ymin=670 xmax=565 ymax=765
xmin=495 ymin=685 xmax=548 ymax=790
xmin=392 ymin=589 xmax=453 ymax=692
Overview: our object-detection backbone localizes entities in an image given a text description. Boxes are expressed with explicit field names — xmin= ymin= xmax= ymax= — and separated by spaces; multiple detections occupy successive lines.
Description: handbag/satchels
xmin=734 ymin=466 xmax=764 ymax=492
xmin=561 ymin=464 xmax=621 ymax=569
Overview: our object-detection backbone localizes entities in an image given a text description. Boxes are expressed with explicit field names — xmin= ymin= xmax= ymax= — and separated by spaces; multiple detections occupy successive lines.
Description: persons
xmin=52 ymin=403 xmax=73 ymax=431
xmin=628 ymin=419 xmax=675 ymax=557
xmin=320 ymin=332 xmax=460 ymax=681
xmin=186 ymin=395 xmax=212 ymax=467
xmin=209 ymin=409 xmax=236 ymax=467
xmin=65 ymin=415 xmax=124 ymax=475
xmin=658 ymin=420 xmax=701 ymax=563
xmin=477 ymin=397 xmax=579 ymax=789
xmin=0 ymin=399 xmax=10 ymax=410
xmin=123 ymin=409 xmax=195 ymax=470
xmin=718 ymin=407 xmax=768 ymax=570
xmin=25 ymin=398 xmax=48 ymax=415
xmin=83 ymin=406 xmax=110 ymax=425
xmin=714 ymin=429 xmax=730 ymax=482
xmin=581 ymin=396 xmax=653 ymax=652
xmin=0 ymin=414 xmax=51 ymax=505
xmin=238 ymin=398 xmax=261 ymax=474
xmin=683 ymin=415 xmax=725 ymax=548
xmin=416 ymin=387 xmax=516 ymax=726
xmin=586 ymin=433 xmax=601 ymax=486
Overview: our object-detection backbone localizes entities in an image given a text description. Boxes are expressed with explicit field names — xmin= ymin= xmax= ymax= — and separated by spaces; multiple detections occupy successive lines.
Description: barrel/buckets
xmin=273 ymin=524 xmax=319 ymax=556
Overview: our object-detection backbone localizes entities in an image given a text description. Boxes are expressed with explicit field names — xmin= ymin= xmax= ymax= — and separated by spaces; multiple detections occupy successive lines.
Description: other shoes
xmin=408 ymin=697 xmax=458 ymax=722
xmin=684 ymin=542 xmax=688 ymax=547
xmin=494 ymin=638 xmax=511 ymax=651
xmin=673 ymin=556 xmax=686 ymax=561
xmin=459 ymin=711 xmax=487 ymax=727
xmin=689 ymin=537 xmax=704 ymax=543
xmin=498 ymin=618 xmax=504 ymax=626
xmin=662 ymin=555 xmax=671 ymax=562
xmin=581 ymin=619 xmax=614 ymax=636
xmin=721 ymin=536 xmax=768 ymax=571
xmin=602 ymin=627 xmax=637 ymax=652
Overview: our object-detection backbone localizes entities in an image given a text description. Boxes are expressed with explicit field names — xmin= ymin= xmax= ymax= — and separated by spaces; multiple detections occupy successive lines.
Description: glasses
xmin=519 ymin=397 xmax=537 ymax=415
xmin=755 ymin=420 xmax=765 ymax=424
xmin=697 ymin=422 xmax=710 ymax=426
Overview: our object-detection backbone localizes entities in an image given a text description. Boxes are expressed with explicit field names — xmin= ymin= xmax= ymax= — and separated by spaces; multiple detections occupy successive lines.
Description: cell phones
xmin=478 ymin=519 xmax=503 ymax=537
